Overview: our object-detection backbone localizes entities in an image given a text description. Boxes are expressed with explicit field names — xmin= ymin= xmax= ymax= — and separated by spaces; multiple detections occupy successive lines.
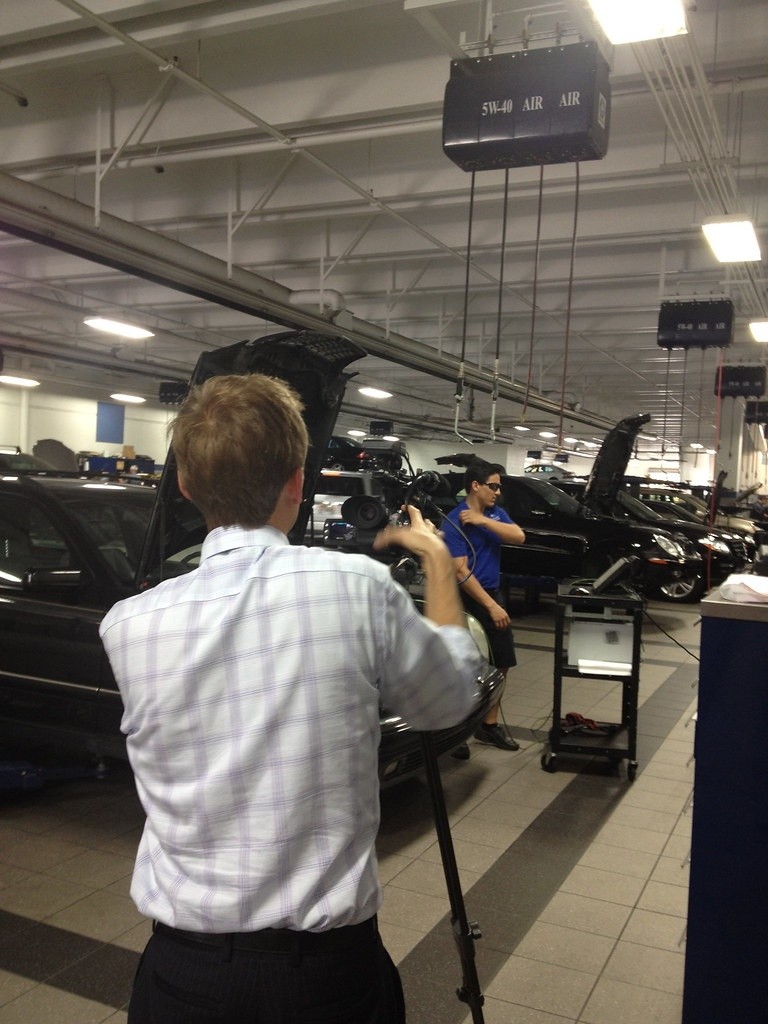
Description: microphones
xmin=418 ymin=469 xmax=451 ymax=497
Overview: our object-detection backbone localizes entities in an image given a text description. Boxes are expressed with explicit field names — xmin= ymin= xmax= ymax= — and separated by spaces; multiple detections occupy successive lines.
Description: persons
xmin=99 ymin=375 xmax=482 ymax=1024
xmin=440 ymin=461 xmax=526 ymax=760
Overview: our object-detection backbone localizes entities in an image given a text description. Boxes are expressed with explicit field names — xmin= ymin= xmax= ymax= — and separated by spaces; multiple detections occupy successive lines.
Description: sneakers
xmin=474 ymin=722 xmax=520 ymax=751
xmin=451 ymin=741 xmax=471 ymax=760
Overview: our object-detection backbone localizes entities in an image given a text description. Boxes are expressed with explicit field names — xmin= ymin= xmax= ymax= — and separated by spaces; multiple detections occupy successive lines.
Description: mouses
xmin=569 ymin=588 xmax=590 ymax=595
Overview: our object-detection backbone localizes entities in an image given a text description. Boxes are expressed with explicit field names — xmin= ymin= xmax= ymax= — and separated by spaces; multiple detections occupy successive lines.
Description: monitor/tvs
xmin=593 ymin=557 xmax=630 ymax=594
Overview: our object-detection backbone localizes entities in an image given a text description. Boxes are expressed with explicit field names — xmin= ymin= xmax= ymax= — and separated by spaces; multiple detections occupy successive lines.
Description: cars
xmin=2 ymin=329 xmax=766 ymax=805
xmin=523 ymin=463 xmax=575 ymax=481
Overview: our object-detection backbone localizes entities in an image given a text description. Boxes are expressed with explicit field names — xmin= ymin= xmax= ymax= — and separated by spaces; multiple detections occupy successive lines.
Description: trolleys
xmin=540 ymin=579 xmax=645 ymax=781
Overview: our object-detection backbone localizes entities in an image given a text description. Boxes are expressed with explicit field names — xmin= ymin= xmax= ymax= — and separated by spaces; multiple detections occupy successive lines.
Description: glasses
xmin=481 ymin=481 xmax=502 ymax=492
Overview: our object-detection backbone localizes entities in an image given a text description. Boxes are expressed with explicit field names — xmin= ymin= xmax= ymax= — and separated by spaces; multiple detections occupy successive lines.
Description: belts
xmin=169 ymin=915 xmax=381 ymax=955
